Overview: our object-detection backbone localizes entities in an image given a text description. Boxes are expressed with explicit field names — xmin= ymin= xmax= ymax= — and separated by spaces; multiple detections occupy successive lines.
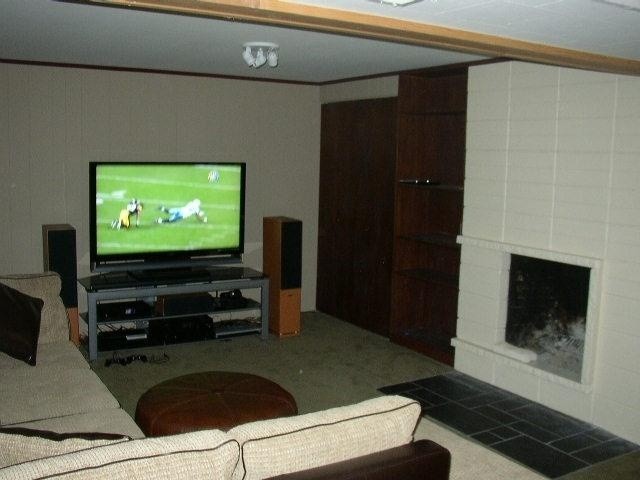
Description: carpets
xmin=75 ymin=312 xmax=450 ymax=433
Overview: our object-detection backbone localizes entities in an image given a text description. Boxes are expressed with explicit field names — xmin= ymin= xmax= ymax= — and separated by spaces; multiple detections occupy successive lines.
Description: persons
xmin=156 ymin=199 xmax=209 ymax=224
xmin=112 ymin=198 xmax=144 ymax=230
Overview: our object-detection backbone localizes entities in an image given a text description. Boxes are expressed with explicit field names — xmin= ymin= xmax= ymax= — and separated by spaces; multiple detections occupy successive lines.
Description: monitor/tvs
xmin=89 ymin=161 xmax=247 ymax=280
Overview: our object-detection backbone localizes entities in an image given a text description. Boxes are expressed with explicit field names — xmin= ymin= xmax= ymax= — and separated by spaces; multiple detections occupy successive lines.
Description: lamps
xmin=241 ymin=41 xmax=282 ymax=70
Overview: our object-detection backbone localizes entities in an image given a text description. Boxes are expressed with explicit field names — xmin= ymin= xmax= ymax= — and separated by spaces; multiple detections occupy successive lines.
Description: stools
xmin=132 ymin=370 xmax=298 ymax=445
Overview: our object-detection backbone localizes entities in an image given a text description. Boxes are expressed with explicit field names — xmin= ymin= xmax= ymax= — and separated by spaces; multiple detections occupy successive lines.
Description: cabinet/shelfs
xmin=388 ymin=65 xmax=476 ymax=367
xmin=78 ymin=265 xmax=270 ymax=363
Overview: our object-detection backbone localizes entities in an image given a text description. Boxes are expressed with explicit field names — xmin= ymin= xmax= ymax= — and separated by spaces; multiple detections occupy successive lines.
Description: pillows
xmin=0 ymin=427 xmax=131 ymax=441
xmin=0 ymin=283 xmax=45 ymax=366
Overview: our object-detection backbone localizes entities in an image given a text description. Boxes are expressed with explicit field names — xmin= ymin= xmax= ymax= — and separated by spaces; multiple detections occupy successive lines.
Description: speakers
xmin=263 ymin=217 xmax=303 ymax=339
xmin=42 ymin=223 xmax=80 ymax=349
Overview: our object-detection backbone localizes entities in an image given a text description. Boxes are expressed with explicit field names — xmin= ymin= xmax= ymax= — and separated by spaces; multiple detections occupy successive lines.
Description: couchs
xmin=0 ymin=271 xmax=451 ymax=479
